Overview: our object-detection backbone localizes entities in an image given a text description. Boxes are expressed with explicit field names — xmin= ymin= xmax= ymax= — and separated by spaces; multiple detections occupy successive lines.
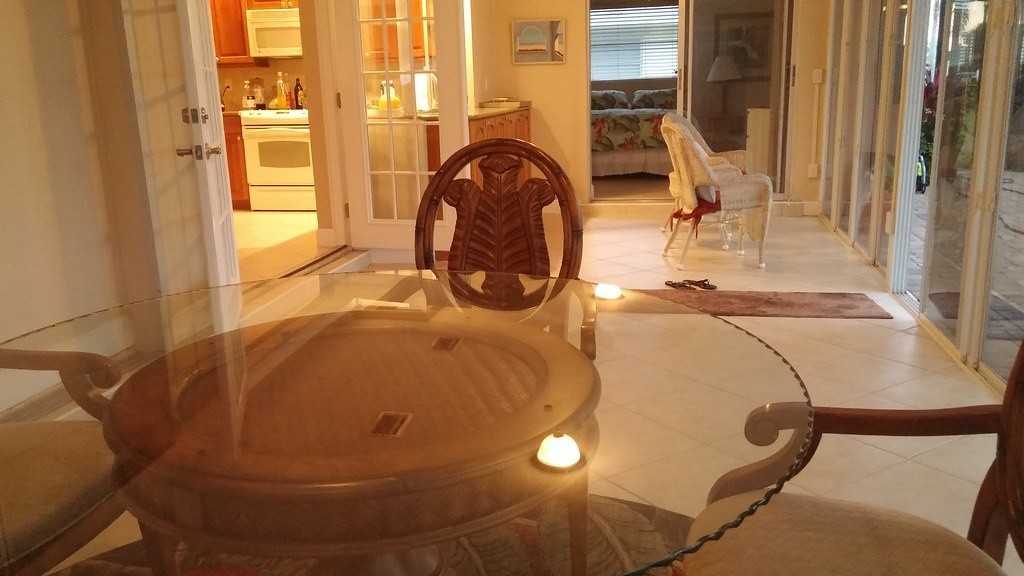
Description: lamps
xmin=531 ymin=430 xmax=586 ymax=473
xmin=707 ymin=56 xmax=742 ymax=114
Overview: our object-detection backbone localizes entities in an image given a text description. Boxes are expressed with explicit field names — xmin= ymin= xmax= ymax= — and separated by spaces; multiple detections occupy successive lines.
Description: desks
xmin=0 ymin=269 xmax=814 ymax=576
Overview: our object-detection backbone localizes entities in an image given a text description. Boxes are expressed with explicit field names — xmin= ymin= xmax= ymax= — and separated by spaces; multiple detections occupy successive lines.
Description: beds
xmin=591 ymin=108 xmax=677 ymax=176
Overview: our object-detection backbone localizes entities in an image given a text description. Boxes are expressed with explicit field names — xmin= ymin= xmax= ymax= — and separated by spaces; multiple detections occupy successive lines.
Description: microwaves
xmin=246 ymin=8 xmax=303 ymax=58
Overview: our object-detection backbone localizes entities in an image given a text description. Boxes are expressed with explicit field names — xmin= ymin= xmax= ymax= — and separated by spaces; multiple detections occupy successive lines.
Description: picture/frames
xmin=511 ymin=19 xmax=566 ymax=65
xmin=714 ymin=11 xmax=773 ymax=83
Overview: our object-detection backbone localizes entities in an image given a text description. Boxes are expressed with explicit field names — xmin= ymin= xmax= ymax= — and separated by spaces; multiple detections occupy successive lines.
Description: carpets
xmin=597 ymin=289 xmax=894 ymax=319
xmin=49 ymin=494 xmax=695 ymax=575
xmin=929 ymin=292 xmax=960 ymax=319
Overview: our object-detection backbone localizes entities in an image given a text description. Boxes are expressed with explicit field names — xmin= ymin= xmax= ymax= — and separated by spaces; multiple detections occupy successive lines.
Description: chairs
xmin=415 ymin=138 xmax=601 ymax=576
xmin=0 ymin=348 xmax=164 ymax=576
xmin=683 ymin=340 xmax=1024 ymax=576
xmin=660 ymin=112 xmax=773 ymax=271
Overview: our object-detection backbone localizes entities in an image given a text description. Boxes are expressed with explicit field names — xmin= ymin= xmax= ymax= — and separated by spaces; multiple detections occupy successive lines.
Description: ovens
xmin=241 ymin=126 xmax=316 ymax=211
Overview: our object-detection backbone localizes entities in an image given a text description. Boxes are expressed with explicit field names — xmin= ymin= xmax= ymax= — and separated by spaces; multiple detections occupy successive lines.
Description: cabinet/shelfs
xmin=247 ymin=0 xmax=299 ymax=10
xmin=372 ymin=0 xmax=436 ymax=59
xmin=426 ymin=110 xmax=531 ymax=220
xmin=209 ymin=0 xmax=255 ymax=64
xmin=223 ymin=115 xmax=250 ymax=210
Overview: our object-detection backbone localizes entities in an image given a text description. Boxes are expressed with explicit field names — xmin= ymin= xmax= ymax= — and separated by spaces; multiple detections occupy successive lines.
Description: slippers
xmin=665 ymin=281 xmax=696 ymax=290
xmin=683 ymin=278 xmax=717 ymax=289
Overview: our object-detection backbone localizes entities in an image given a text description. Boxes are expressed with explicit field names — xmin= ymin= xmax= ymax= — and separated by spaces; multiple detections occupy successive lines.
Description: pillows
xmin=591 ymin=90 xmax=628 ymax=110
xmin=631 ymin=88 xmax=677 ymax=109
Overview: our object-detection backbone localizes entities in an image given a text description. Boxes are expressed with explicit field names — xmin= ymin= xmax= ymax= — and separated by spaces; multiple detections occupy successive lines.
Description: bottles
xmin=242 ymin=71 xmax=303 ymax=110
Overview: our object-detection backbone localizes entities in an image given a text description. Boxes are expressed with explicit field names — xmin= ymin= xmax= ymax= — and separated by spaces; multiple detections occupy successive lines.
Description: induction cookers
xmin=238 ymin=106 xmax=309 ymax=125
